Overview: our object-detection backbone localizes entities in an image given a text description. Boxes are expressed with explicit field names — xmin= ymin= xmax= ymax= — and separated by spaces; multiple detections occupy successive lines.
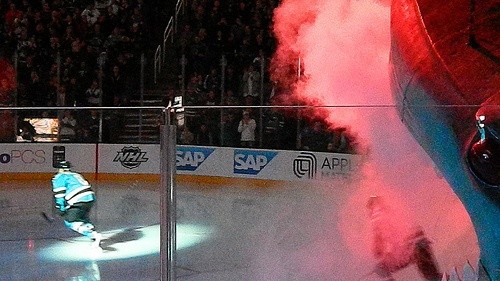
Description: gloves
xmin=56 ymin=210 xmax=66 ymax=216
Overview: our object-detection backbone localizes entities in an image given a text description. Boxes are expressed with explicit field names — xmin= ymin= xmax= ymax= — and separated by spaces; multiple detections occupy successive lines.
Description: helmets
xmin=56 ymin=160 xmax=71 ymax=168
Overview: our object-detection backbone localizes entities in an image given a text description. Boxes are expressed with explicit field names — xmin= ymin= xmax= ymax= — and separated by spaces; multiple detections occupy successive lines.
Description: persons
xmin=300 ymin=119 xmax=349 ymax=153
xmin=238 ymin=111 xmax=256 ymax=148
xmin=51 ymin=160 xmax=106 ymax=249
xmin=0 ymin=0 xmax=284 ymax=148
